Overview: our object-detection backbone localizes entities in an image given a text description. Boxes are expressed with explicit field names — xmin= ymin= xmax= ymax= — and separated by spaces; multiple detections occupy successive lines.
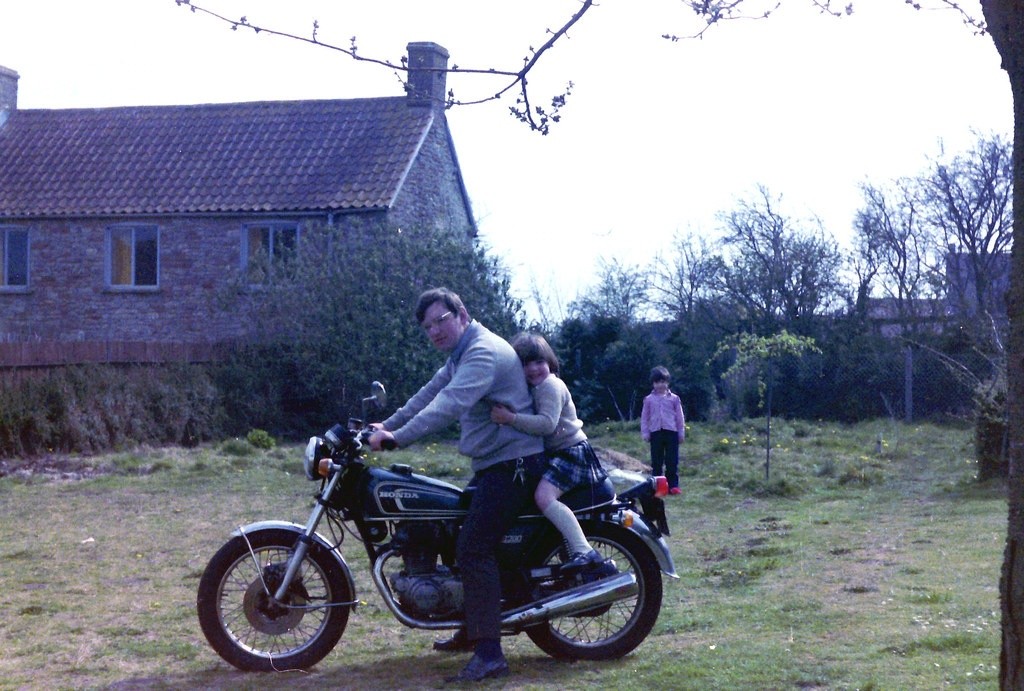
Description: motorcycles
xmin=196 ymin=380 xmax=681 ymax=674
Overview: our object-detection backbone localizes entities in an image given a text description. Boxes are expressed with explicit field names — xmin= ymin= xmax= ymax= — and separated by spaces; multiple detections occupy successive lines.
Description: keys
xmin=513 ymin=457 xmax=528 ymax=490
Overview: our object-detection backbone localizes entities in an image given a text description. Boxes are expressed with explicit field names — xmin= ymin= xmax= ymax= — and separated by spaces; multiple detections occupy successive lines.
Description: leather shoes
xmin=559 ymin=549 xmax=605 ymax=576
xmin=433 ymin=629 xmax=478 ymax=653
xmin=445 ymin=654 xmax=510 ymax=685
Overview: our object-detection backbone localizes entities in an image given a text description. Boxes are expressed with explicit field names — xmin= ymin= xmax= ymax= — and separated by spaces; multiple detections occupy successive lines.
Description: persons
xmin=641 ymin=366 xmax=687 ymax=497
xmin=369 ymin=286 xmax=553 ymax=685
xmin=491 ymin=333 xmax=612 ymax=571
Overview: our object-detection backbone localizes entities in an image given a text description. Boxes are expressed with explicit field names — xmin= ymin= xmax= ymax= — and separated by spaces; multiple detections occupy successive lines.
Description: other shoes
xmin=669 ymin=487 xmax=681 ymax=494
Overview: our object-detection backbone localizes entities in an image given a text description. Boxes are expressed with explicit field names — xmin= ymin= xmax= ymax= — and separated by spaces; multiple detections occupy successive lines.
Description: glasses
xmin=421 ymin=311 xmax=453 ymax=333
